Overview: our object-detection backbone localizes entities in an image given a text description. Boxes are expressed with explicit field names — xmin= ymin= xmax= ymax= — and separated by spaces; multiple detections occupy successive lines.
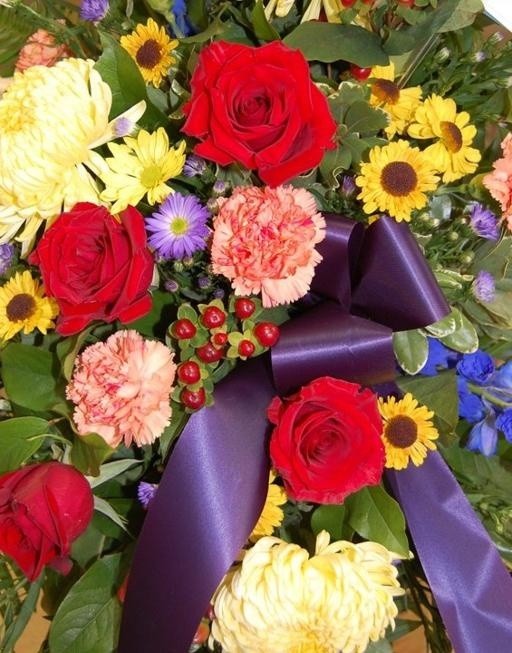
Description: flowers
xmin=0 ymin=0 xmax=512 ymax=653
